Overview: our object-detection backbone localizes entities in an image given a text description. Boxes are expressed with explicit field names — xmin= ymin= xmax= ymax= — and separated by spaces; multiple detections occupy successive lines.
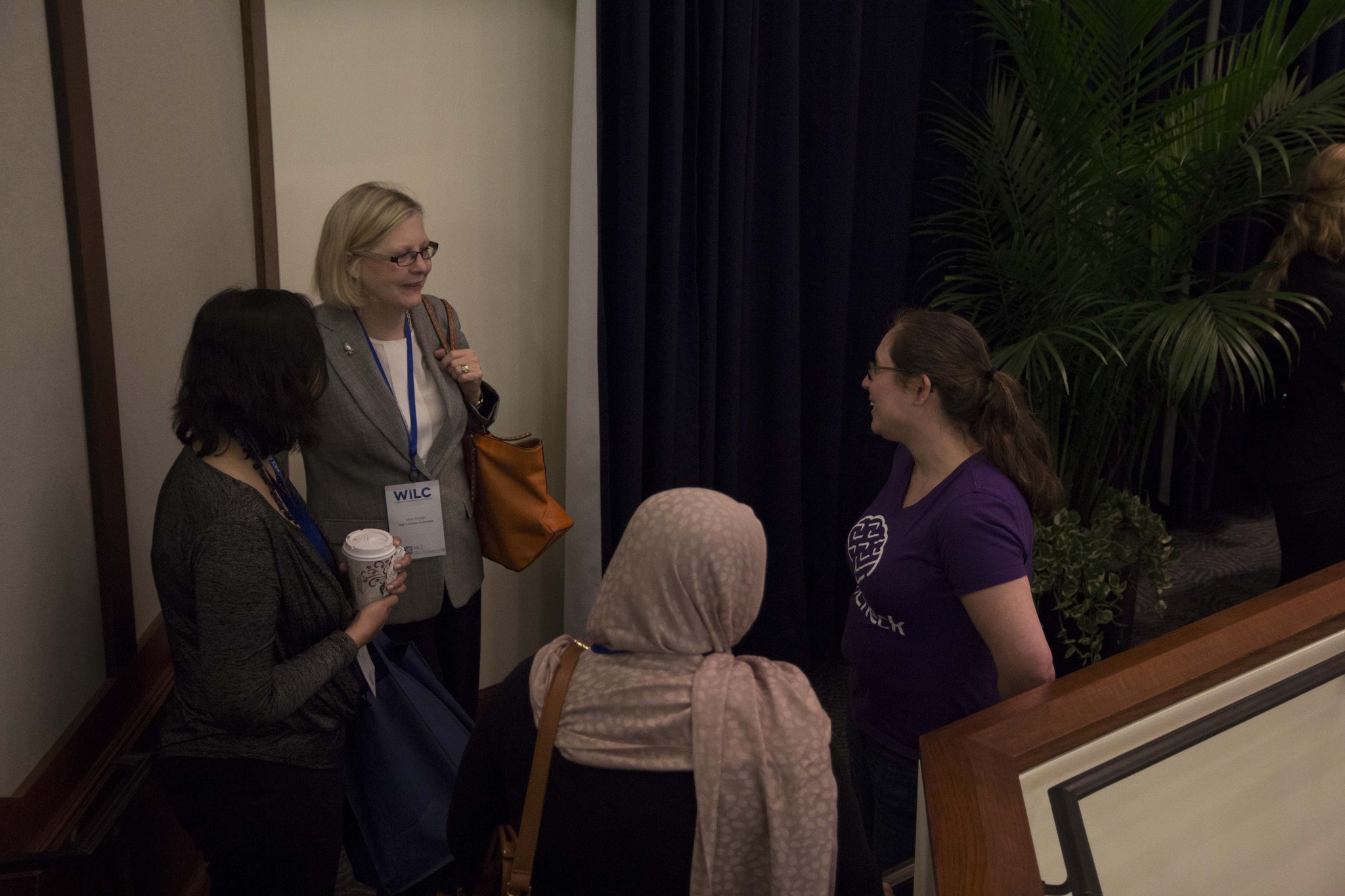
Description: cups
xmin=340 ymin=528 xmax=398 ymax=612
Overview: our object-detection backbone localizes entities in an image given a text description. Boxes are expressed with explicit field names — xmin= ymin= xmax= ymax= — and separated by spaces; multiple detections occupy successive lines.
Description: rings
xmin=461 ymin=365 xmax=469 ymax=373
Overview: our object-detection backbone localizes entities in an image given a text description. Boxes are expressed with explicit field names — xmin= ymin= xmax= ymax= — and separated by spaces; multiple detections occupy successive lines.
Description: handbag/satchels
xmin=335 ymin=629 xmax=477 ymax=896
xmin=479 ymin=822 xmax=531 ymax=896
xmin=462 ymin=422 xmax=576 ymax=574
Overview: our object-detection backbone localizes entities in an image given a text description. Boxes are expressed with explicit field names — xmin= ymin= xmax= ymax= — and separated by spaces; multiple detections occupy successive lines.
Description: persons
xmin=843 ymin=312 xmax=1058 ymax=865
xmin=1249 ymin=143 xmax=1345 ymax=591
xmin=443 ymin=488 xmax=896 ymax=896
xmin=149 ymin=288 xmax=412 ymax=896
xmin=296 ymin=183 xmax=500 ymax=729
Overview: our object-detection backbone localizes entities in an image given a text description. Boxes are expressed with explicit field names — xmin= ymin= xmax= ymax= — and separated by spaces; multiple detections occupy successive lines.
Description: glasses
xmin=868 ymin=358 xmax=935 ymax=393
xmin=352 ymin=241 xmax=438 ymax=266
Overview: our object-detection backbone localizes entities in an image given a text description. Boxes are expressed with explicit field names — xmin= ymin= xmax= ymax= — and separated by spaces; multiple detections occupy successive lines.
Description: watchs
xmin=474 ymin=393 xmax=484 ymax=409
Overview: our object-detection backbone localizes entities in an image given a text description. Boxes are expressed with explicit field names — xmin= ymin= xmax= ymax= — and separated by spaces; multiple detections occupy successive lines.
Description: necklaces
xmin=406 ymin=310 xmax=412 ymax=331
xmin=240 ymin=443 xmax=293 ymax=522
